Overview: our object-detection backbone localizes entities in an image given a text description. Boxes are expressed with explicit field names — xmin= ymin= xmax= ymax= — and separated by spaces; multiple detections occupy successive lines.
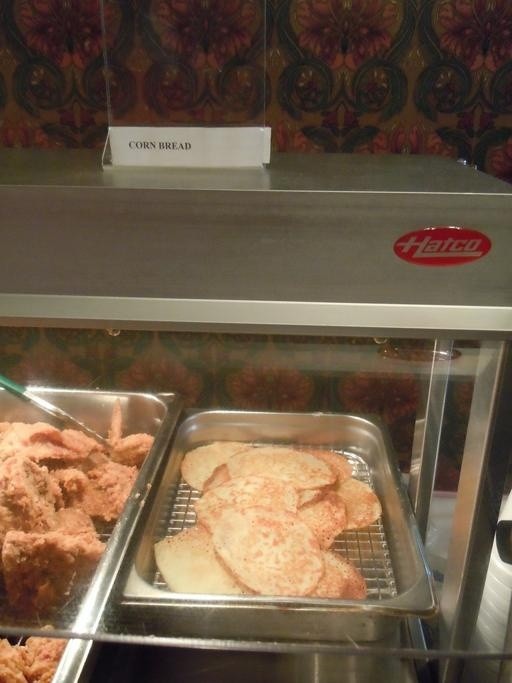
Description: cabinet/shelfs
xmin=0 ymin=146 xmax=512 ymax=683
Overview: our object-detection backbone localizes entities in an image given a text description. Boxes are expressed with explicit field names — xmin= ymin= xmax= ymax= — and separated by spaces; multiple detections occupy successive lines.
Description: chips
xmin=154 ymin=439 xmax=383 ymax=599
